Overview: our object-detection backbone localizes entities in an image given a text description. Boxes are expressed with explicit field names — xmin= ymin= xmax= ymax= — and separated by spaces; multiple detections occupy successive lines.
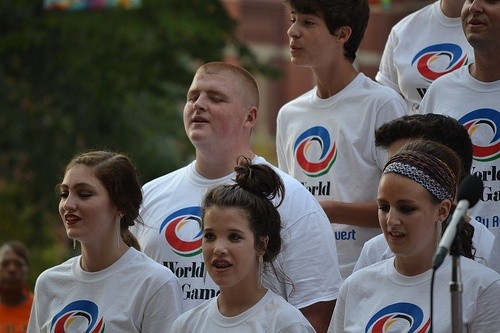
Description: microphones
xmin=431 ymin=175 xmax=483 ymax=269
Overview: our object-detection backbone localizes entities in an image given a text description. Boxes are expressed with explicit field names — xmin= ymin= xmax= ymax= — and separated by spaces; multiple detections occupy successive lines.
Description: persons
xmin=327 ymin=140 xmax=500 ymax=333
xmin=416 ymin=0 xmax=500 ymax=237
xmin=276 ymin=0 xmax=407 ymax=279
xmin=27 ymin=151 xmax=185 ymax=333
xmin=128 ymin=63 xmax=344 ymax=333
xmin=354 ymin=113 xmax=495 ymax=271
xmin=375 ymin=0 xmax=475 ymax=114
xmin=0 ymin=240 xmax=34 ymax=333
xmin=168 ymin=163 xmax=316 ymax=333
